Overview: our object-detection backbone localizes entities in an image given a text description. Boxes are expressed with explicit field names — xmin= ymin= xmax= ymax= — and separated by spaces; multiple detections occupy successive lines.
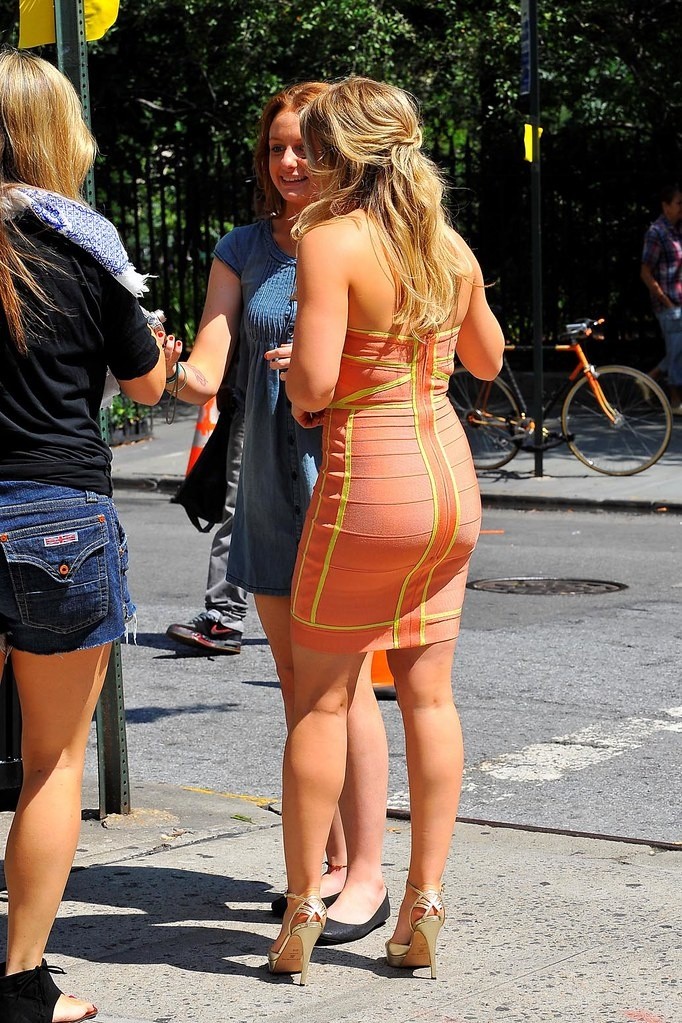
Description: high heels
xmin=385 ymin=880 xmax=446 ymax=980
xmin=266 ymin=890 xmax=328 ymax=985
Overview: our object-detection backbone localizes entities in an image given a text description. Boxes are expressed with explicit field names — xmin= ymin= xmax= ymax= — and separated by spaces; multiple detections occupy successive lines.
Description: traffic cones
xmin=186 ymin=392 xmax=221 ymax=485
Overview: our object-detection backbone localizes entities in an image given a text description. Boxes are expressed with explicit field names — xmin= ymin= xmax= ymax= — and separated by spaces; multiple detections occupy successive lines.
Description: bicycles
xmin=446 ymin=316 xmax=674 ymax=477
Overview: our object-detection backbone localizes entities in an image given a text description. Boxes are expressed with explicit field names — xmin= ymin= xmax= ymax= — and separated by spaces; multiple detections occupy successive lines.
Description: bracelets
xmin=167 ymin=365 xmax=188 ymax=392
xmin=166 ymin=362 xmax=180 ymax=383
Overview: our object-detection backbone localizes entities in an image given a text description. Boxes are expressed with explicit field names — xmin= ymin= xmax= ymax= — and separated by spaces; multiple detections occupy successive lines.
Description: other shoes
xmin=670 ymin=404 xmax=682 ymax=415
xmin=0 ymin=958 xmax=98 ymax=1023
xmin=636 ymin=378 xmax=652 ymax=405
xmin=319 ymin=886 xmax=390 ymax=942
xmin=271 ymin=892 xmax=340 ymax=915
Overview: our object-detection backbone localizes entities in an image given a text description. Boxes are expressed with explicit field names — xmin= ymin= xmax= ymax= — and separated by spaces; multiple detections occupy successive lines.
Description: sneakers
xmin=165 ymin=611 xmax=242 ymax=652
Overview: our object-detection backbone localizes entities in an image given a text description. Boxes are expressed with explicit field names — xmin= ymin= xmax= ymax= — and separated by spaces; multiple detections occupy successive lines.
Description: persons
xmin=148 ymin=82 xmax=390 ymax=944
xmin=269 ymin=79 xmax=504 ymax=987
xmin=0 ymin=48 xmax=166 ymax=1023
xmin=635 ymin=184 xmax=682 ymax=414
xmin=164 ymin=177 xmax=288 ymax=653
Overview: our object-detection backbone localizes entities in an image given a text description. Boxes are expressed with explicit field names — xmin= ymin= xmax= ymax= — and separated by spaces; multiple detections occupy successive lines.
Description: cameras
xmin=139 ymin=305 xmax=167 ymax=344
